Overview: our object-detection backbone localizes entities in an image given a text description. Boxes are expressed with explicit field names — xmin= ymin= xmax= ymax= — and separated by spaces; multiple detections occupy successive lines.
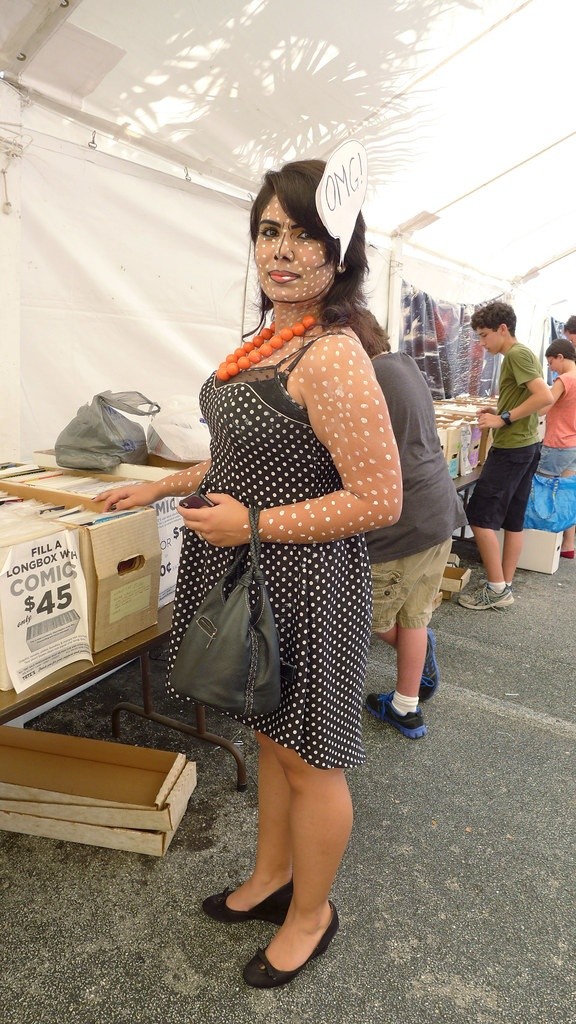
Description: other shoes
xmin=561 ymin=549 xmax=575 ymax=559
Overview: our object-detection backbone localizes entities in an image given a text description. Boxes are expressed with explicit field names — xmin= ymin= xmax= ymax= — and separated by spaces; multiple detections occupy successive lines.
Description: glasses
xmin=546 ymin=358 xmax=555 ymax=366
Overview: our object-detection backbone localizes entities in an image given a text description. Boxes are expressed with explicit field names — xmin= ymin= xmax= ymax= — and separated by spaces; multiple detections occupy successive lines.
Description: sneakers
xmin=365 ymin=692 xmax=428 ymax=740
xmin=457 ymin=582 xmax=514 ymax=610
xmin=477 ymin=578 xmax=514 ymax=594
xmin=418 ymin=628 xmax=439 ymax=702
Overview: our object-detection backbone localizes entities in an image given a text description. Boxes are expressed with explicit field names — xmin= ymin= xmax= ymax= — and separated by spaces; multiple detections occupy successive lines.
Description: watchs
xmin=501 ymin=411 xmax=512 ymax=425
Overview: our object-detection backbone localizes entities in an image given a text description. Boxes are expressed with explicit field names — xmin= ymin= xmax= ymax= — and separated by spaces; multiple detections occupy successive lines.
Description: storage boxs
xmin=1 ymin=505 xmax=84 ymax=693
xmin=1 ymin=480 xmax=161 ymax=657
xmin=498 ymin=526 xmax=564 ymax=574
xmin=435 ymin=391 xmax=552 ymax=481
xmin=0 ymin=725 xmax=199 ymax=857
xmin=2 ymin=449 xmax=190 ymax=612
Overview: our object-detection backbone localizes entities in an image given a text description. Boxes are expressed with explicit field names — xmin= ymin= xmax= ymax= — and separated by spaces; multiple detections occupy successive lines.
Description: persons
xmin=346 ymin=306 xmax=470 ymax=738
xmin=457 ymin=302 xmax=555 ymax=611
xmin=563 ymin=315 xmax=576 ymax=346
xmin=534 ymin=339 xmax=576 ymax=559
xmin=90 ymin=159 xmax=403 ymax=989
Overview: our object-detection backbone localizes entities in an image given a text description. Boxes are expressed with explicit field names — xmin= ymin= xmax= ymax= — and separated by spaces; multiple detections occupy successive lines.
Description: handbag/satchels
xmin=169 ymin=556 xmax=283 ymax=719
xmin=53 ymin=388 xmax=212 ymax=472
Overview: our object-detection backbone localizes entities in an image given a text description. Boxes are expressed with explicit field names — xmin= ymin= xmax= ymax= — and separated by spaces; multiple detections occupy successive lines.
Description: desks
xmin=0 ymin=599 xmax=248 ymax=793
xmin=448 ymin=464 xmax=485 ymax=545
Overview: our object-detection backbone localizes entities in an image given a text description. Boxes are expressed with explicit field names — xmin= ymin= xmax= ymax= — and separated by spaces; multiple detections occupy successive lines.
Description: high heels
xmin=202 ymin=872 xmax=295 ymax=925
xmin=242 ymin=900 xmax=339 ymax=988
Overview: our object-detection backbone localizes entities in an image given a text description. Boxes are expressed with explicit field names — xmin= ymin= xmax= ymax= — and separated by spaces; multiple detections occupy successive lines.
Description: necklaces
xmin=216 ymin=315 xmax=316 ymax=381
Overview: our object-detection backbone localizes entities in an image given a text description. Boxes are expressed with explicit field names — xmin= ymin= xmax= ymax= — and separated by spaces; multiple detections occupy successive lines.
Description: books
xmin=0 ymin=461 xmax=154 ymax=552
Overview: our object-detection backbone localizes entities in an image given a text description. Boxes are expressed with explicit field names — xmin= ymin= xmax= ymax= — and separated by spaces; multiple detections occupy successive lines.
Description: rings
xmin=199 ymin=531 xmax=204 ymax=541
